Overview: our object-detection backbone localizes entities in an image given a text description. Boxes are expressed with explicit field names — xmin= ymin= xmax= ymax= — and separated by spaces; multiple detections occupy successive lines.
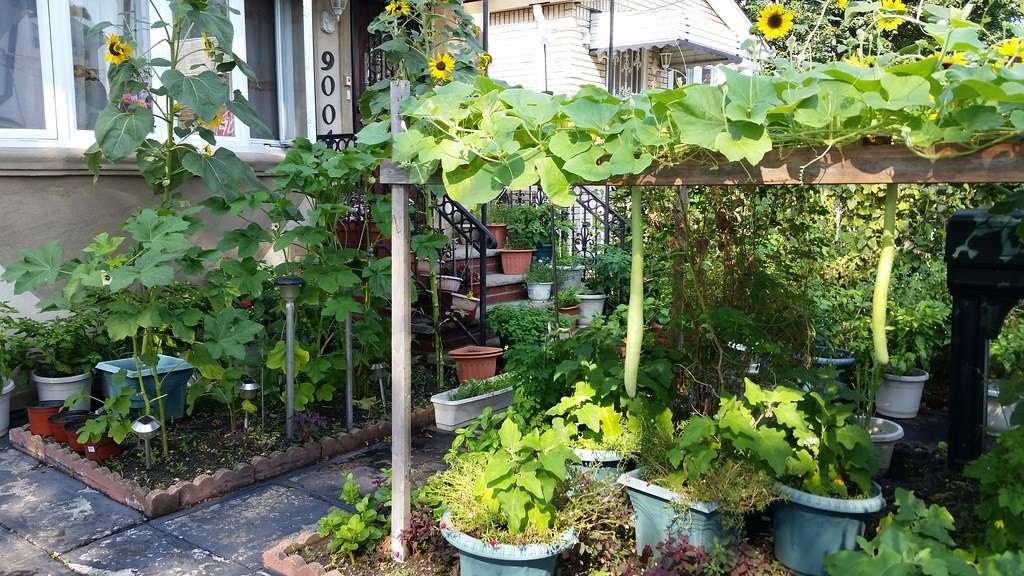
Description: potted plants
xmin=987 ymin=319 xmax=1024 ymax=438
xmin=423 ymin=403 xmax=634 ymax=576
xmin=417 ymin=202 xmax=635 ymax=332
xmin=0 ymin=301 xmax=98 ymax=440
xmin=564 ymin=263 xmax=957 ymax=576
xmin=430 ymin=372 xmax=528 ymax=429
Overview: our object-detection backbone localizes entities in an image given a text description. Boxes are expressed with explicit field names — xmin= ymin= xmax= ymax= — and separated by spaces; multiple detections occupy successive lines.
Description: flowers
xmin=440 ymin=265 xmax=479 ymax=285
xmin=466 ymin=291 xmax=474 ymax=299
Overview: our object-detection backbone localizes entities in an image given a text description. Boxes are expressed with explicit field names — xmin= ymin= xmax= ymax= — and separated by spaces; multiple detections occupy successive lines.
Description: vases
xmin=451 ymin=293 xmax=480 ymax=315
xmin=442 ymin=284 xmax=480 ymax=321
xmin=84 ymin=434 xmax=124 ymax=461
xmin=96 ymin=355 xmax=195 ymax=423
xmin=49 ymin=410 xmax=91 ymax=442
xmin=449 ymin=344 xmax=503 ymax=383
xmin=64 ymin=415 xmax=100 ymax=452
xmin=24 ymin=399 xmax=70 ymax=435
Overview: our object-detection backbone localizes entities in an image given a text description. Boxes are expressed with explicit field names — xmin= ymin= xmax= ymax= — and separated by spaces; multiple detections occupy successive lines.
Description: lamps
xmin=657 ymin=45 xmax=674 ymax=71
xmin=237 ymin=378 xmax=261 ymax=428
xmin=321 ymin=0 xmax=348 ymax=33
xmin=130 ymin=414 xmax=160 ymax=468
xmin=369 ymin=358 xmax=390 ymax=416
xmin=275 ymin=274 xmax=303 ymax=438
